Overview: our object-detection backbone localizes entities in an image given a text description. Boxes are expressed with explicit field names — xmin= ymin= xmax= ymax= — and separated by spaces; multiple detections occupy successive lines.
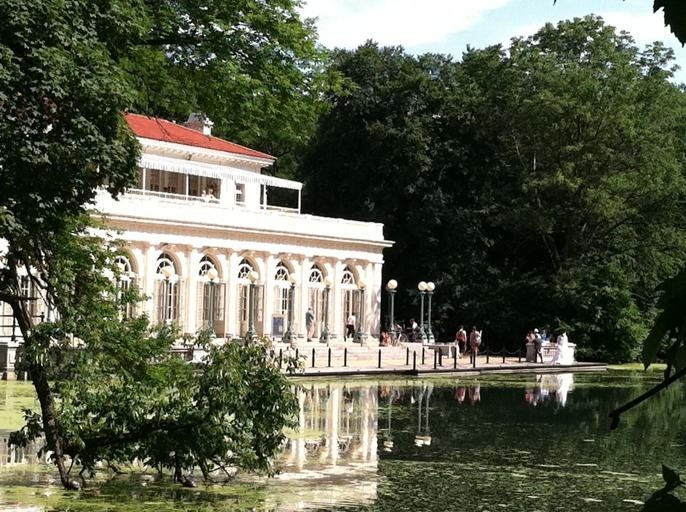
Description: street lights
xmin=324 ymin=275 xmax=334 ymax=347
xmin=388 ymin=279 xmax=398 ymax=332
xmin=282 ymin=273 xmax=300 ymax=343
xmin=247 ymin=271 xmax=259 ymax=336
xmin=358 ymin=278 xmax=367 ymax=347
xmin=207 ymin=268 xmax=218 ymax=328
xmin=417 ymin=281 xmax=435 ymax=339
xmin=163 ymin=266 xmax=172 ymax=329
xmin=383 ymin=397 xmax=393 ymax=448
xmin=414 ymin=390 xmax=432 ymax=445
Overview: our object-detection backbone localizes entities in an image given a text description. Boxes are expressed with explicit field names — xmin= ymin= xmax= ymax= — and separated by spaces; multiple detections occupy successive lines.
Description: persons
xmin=298 ymin=373 xmax=575 ymax=417
xmin=304 ymin=307 xmax=316 ymax=342
xmin=201 ymin=189 xmax=207 ymax=203
xmin=520 ymin=324 xmax=557 ymax=363
xmin=346 ymin=311 xmax=356 ymax=338
xmin=456 ymin=324 xmax=483 ymax=359
xmin=207 ymin=187 xmax=216 ymax=203
xmin=379 ymin=316 xmax=419 ymax=346
